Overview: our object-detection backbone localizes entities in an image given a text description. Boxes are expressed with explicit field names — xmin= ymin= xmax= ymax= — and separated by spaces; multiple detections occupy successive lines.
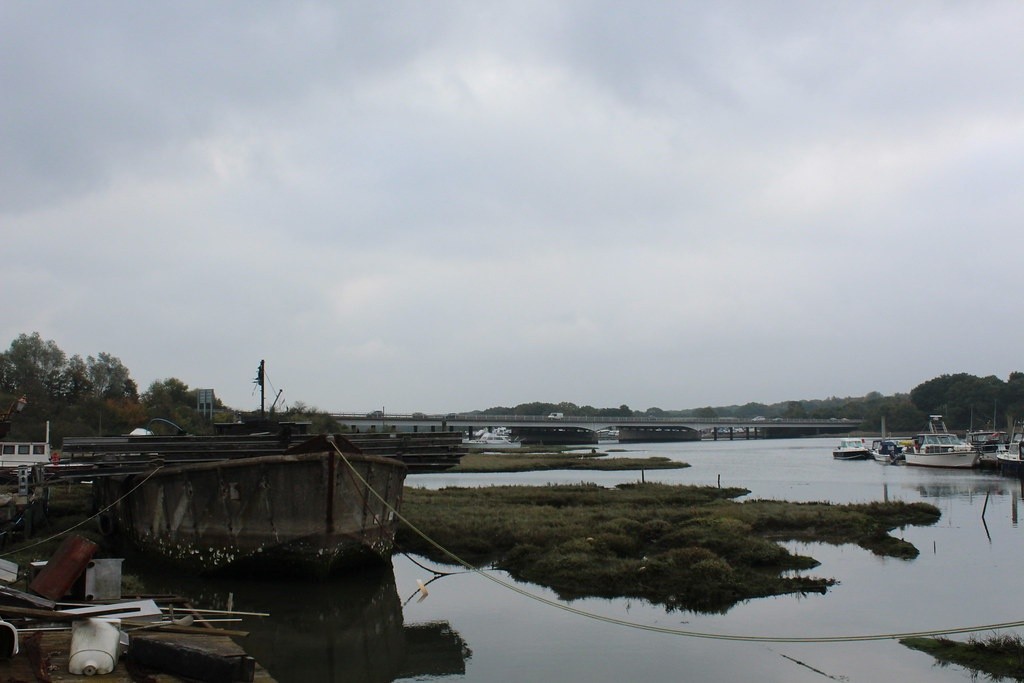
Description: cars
xmin=412 ymin=413 xmax=428 ymax=419
xmin=366 ymin=411 xmax=383 ymax=419
xmin=751 ymin=416 xmax=765 ymax=422
xmin=772 ymin=418 xmax=784 ymax=422
xmin=445 ymin=413 xmax=458 ymax=420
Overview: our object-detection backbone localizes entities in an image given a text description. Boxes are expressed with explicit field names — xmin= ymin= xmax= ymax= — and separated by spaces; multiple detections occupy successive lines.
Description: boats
xmin=871 ymin=398 xmax=1024 ymax=470
xmin=459 ymin=433 xmax=522 ymax=449
xmin=832 ymin=438 xmax=870 ymax=459
xmin=87 ymin=433 xmax=410 ymax=580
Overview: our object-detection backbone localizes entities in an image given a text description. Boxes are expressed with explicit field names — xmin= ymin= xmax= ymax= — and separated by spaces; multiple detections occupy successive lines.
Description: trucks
xmin=548 ymin=412 xmax=564 ymax=421
